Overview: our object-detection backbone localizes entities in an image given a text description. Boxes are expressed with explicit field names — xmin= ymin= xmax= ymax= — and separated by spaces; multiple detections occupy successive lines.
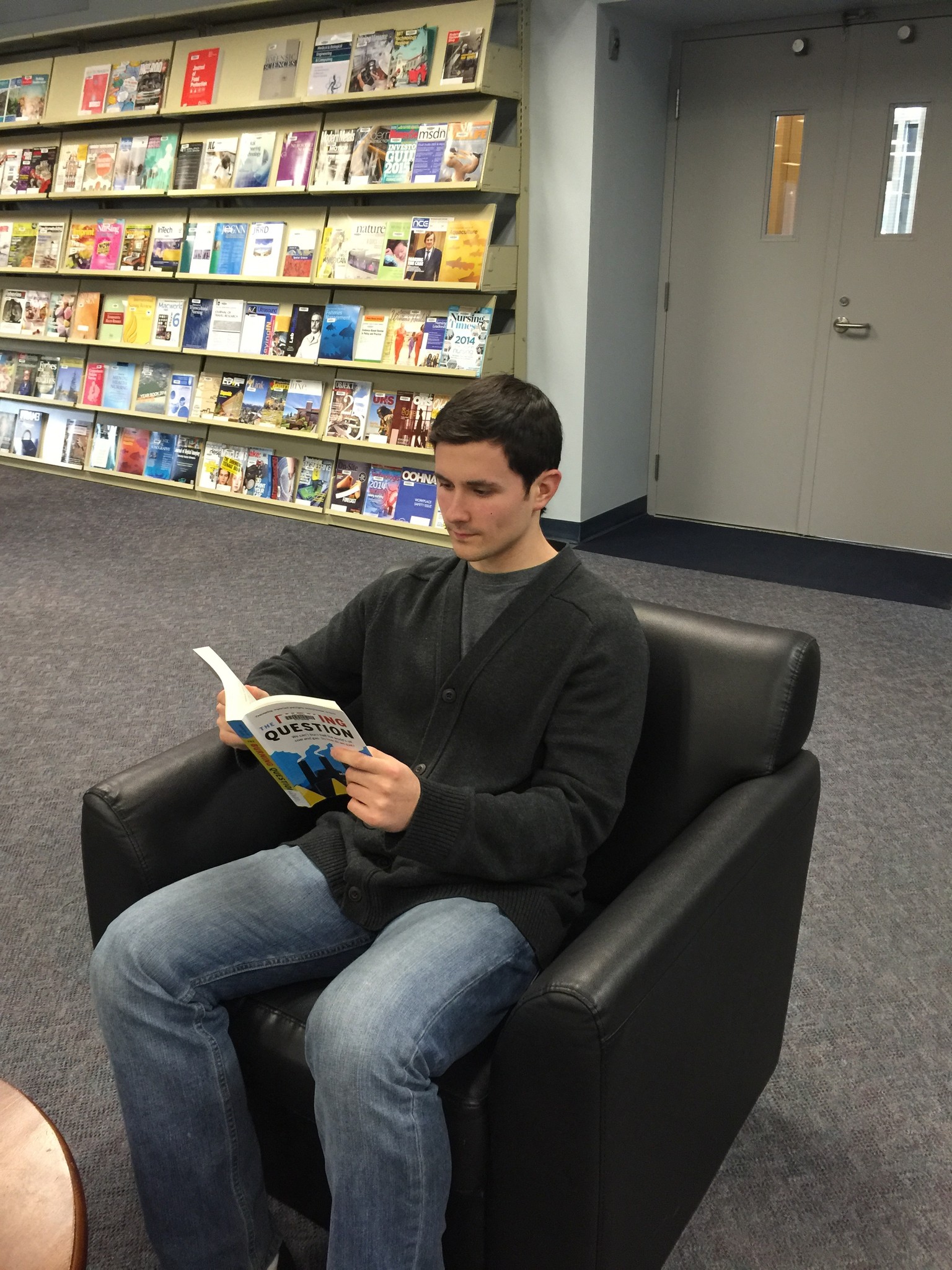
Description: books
xmin=193 ymin=647 xmax=371 ymax=808
xmin=0 ymin=24 xmax=492 ymax=531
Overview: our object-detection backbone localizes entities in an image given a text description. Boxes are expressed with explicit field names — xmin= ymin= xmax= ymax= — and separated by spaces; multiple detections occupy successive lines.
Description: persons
xmin=426 ymin=354 xmax=432 ymax=367
xmin=295 ymin=311 xmax=323 ymax=360
xmin=17 ymin=369 xmax=32 ymax=396
xmin=405 ymin=232 xmax=442 ymax=281
xmin=385 ymin=240 xmax=408 ymax=262
xmin=357 ymin=39 xmax=393 ymax=91
xmin=272 ymin=345 xmax=287 ymax=356
xmin=271 ymin=332 xmax=288 ymax=343
xmin=215 ymin=468 xmax=230 ymax=490
xmin=431 ymin=355 xmax=439 ymax=367
xmin=90 ymin=375 xmax=650 ymax=1270
xmin=227 ymin=467 xmax=243 ymax=493
xmin=435 ymin=354 xmax=440 ymax=367
xmin=414 ymin=324 xmax=425 ymax=366
xmin=394 ymin=323 xmax=406 ymax=365
xmin=408 ymin=332 xmax=416 ymax=358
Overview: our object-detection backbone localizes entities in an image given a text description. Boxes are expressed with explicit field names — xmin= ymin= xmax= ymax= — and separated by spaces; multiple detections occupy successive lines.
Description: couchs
xmin=81 ymin=564 xmax=821 ymax=1270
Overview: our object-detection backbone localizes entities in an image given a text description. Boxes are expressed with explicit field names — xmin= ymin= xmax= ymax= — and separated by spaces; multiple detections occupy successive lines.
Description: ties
xmin=423 ymin=251 xmax=430 ymax=270
xmin=311 ymin=336 xmax=314 ymax=343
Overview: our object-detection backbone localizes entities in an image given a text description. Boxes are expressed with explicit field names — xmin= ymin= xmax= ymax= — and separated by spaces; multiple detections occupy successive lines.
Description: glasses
xmin=24 ymin=373 xmax=30 ymax=377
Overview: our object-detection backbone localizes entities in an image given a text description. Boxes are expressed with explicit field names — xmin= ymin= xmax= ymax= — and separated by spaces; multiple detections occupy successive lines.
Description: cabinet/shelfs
xmin=0 ymin=0 xmax=529 ymax=550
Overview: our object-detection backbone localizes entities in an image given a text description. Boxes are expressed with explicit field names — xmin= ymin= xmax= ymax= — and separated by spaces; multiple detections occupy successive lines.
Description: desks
xmin=0 ymin=1079 xmax=90 ymax=1270
